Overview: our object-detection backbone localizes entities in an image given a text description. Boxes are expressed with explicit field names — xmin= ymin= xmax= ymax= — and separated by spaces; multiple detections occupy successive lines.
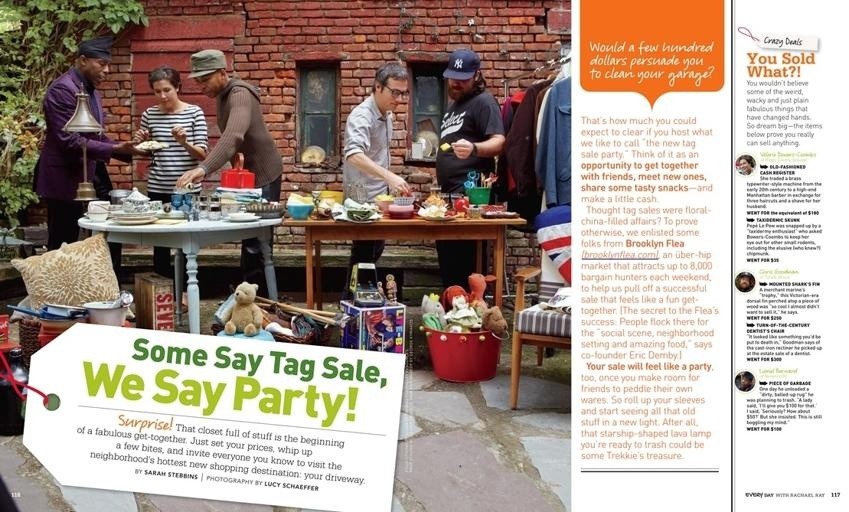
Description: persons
xmin=737 ymin=371 xmax=755 ymax=393
xmin=175 ymin=48 xmax=284 ymax=301
xmin=735 ymin=155 xmax=756 ymax=177
xmin=734 ymin=272 xmax=755 ymax=292
xmin=133 ymin=64 xmax=209 ymax=301
xmin=433 ymin=48 xmax=506 ymax=294
xmin=32 ymin=38 xmax=145 ymax=290
xmin=339 ymin=61 xmax=413 ymax=302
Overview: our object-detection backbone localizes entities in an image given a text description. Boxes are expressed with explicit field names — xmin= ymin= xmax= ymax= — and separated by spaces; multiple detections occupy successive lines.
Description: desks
xmin=283 ymin=209 xmax=530 ymax=313
xmin=77 ymin=215 xmax=284 ymax=335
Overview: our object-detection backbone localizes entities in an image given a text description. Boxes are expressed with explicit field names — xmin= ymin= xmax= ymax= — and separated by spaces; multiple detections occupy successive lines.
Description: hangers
xmin=528 ymin=60 xmax=569 ymax=81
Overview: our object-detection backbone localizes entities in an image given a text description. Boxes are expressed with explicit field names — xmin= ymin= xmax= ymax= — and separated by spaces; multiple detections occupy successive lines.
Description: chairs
xmin=508 ymin=247 xmax=571 ymax=411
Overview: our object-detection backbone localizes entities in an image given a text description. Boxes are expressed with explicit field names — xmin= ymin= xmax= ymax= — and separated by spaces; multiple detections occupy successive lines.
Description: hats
xmin=78 ymin=36 xmax=112 ymax=61
xmin=442 ymin=48 xmax=481 ymax=82
xmin=187 ymin=49 xmax=227 ymax=79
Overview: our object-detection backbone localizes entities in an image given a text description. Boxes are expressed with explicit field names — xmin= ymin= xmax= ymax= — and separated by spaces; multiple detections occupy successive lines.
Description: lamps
xmin=59 ymin=80 xmax=105 ymax=212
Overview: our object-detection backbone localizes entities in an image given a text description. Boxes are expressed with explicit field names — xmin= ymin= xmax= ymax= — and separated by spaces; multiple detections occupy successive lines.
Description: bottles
xmin=210 ymin=197 xmax=221 ymax=219
xmin=468 ymin=208 xmax=519 ymax=219
xmin=197 ymin=195 xmax=208 ymax=217
xmin=0 ymin=348 xmax=29 ymax=434
xmin=86 ymin=187 xmax=162 ymax=224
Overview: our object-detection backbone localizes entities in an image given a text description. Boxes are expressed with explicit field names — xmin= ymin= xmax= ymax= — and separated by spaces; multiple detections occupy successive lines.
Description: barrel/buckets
xmin=418 ymin=319 xmax=511 ymax=384
xmin=466 ymin=188 xmax=491 ymax=206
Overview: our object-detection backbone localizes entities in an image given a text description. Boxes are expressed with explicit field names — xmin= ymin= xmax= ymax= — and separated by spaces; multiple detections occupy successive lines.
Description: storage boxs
xmin=337 ymin=298 xmax=407 ymax=354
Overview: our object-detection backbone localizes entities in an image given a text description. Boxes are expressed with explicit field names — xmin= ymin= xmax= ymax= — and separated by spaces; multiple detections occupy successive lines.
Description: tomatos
xmin=454 ymin=197 xmax=469 ymax=212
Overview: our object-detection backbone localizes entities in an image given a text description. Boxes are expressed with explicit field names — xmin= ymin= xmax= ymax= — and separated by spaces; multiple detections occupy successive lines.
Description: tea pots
xmin=220 ymin=153 xmax=255 ymax=188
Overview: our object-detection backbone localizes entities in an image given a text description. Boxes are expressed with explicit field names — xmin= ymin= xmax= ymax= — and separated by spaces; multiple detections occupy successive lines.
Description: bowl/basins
xmin=315 ymin=190 xmax=344 ymax=205
xmin=246 ymin=203 xmax=285 ymax=219
xmin=467 ymin=187 xmax=490 ymax=205
xmin=222 ymin=202 xmax=242 ymax=213
xmin=286 ymin=205 xmax=314 ymax=219
xmin=85 ymin=300 xmax=130 ymax=326
xmin=419 ymin=326 xmax=500 ymax=382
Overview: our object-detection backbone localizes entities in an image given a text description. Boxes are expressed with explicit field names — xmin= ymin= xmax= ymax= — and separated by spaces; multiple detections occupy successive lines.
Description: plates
xmin=415 ymin=131 xmax=439 ymax=158
xmin=417 ymin=136 xmax=433 ymax=158
xmin=302 ymin=145 xmax=327 ymax=163
xmin=159 ymin=211 xmax=192 ymax=220
xmin=226 ymin=211 xmax=261 ymax=222
xmin=373 ymin=192 xmax=464 ymax=221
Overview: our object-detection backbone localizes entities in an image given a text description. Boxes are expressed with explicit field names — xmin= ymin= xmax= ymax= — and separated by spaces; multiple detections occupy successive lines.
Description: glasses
xmin=381 ymin=83 xmax=411 ymax=99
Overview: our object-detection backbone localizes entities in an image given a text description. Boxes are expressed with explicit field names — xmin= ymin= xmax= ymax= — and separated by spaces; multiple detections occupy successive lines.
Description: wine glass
xmin=185 ymin=194 xmax=194 ymax=211
xmin=140 ymin=146 xmax=168 ymax=168
xmin=171 ymin=195 xmax=183 ymax=210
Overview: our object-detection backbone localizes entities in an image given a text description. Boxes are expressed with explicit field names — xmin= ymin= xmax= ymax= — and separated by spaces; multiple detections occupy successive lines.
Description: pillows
xmin=7 ymin=232 xmax=121 ymax=315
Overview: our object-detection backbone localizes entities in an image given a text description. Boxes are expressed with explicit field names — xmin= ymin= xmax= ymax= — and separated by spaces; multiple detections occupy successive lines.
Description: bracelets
xmin=471 ymin=143 xmax=478 ymax=157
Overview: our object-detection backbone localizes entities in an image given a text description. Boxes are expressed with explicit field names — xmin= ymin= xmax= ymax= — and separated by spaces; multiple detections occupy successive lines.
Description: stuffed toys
xmin=421 ymin=273 xmax=509 ymax=335
xmin=219 ymin=281 xmax=263 ymax=336
